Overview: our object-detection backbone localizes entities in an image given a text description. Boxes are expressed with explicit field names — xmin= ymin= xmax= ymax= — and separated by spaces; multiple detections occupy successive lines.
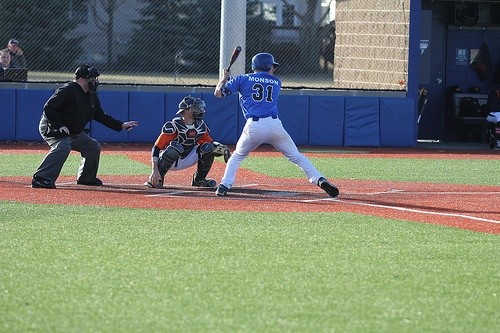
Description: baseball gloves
xmin=210 ymin=141 xmax=231 ymax=164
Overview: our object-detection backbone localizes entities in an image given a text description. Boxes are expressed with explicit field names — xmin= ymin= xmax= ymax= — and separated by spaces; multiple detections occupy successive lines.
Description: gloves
xmin=213 ymin=144 xmax=230 ymax=163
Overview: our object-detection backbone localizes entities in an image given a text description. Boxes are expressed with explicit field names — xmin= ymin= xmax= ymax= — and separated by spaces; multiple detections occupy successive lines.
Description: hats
xmin=175 ymin=95 xmax=197 ymax=115
xmin=7 ymin=39 xmax=18 ymax=44
xmin=74 ymin=64 xmax=101 ymax=79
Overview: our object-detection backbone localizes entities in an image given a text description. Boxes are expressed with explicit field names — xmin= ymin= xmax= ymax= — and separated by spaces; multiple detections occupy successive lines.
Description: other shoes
xmin=191 ymin=175 xmax=216 ymax=187
xmin=143 ymin=179 xmax=164 ymax=189
xmin=318 ymin=177 xmax=339 ymax=198
xmin=32 ymin=175 xmax=56 ymax=189
xmin=76 ymin=176 xmax=103 ymax=186
xmin=215 ymin=184 xmax=229 ymax=196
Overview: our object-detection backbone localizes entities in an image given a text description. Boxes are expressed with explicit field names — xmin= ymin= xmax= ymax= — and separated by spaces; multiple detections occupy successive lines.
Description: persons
xmin=0 ymin=38 xmax=26 ymax=80
xmin=213 ymin=53 xmax=340 ymax=198
xmin=143 ymin=97 xmax=231 ymax=188
xmin=31 ymin=65 xmax=139 ymax=189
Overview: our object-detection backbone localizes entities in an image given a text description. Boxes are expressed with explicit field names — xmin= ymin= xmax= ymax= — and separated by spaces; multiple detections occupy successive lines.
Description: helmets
xmin=251 ymin=53 xmax=280 ymax=70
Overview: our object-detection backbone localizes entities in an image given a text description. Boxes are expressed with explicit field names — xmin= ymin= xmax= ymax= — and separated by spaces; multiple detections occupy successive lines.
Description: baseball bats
xmin=226 ymin=46 xmax=242 ymax=72
